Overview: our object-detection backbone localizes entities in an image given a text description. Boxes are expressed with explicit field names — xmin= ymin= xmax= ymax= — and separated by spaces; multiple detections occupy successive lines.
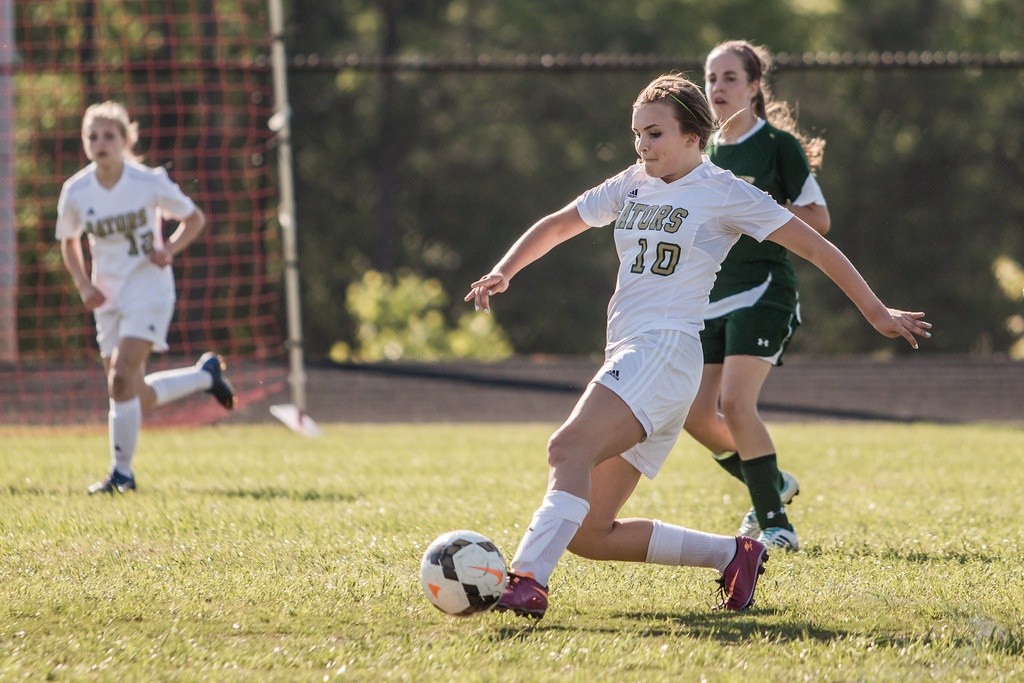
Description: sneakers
xmin=196 ymin=352 xmax=235 ymax=410
xmin=710 ymin=536 xmax=770 ymax=610
xmin=756 ymin=523 xmax=799 ymax=552
xmin=490 ymin=572 xmax=549 ymax=619
xmin=738 ymin=471 xmax=800 ymax=540
xmin=87 ymin=468 xmax=136 ymax=496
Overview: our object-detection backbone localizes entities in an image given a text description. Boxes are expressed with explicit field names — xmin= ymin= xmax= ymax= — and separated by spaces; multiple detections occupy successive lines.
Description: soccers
xmin=419 ymin=530 xmax=506 ymax=616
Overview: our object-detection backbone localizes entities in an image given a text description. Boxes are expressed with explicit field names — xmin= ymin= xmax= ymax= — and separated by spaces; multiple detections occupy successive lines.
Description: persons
xmin=53 ymin=103 xmax=235 ymax=496
xmin=680 ymin=41 xmax=830 ymax=556
xmin=463 ymin=75 xmax=934 ymax=621
